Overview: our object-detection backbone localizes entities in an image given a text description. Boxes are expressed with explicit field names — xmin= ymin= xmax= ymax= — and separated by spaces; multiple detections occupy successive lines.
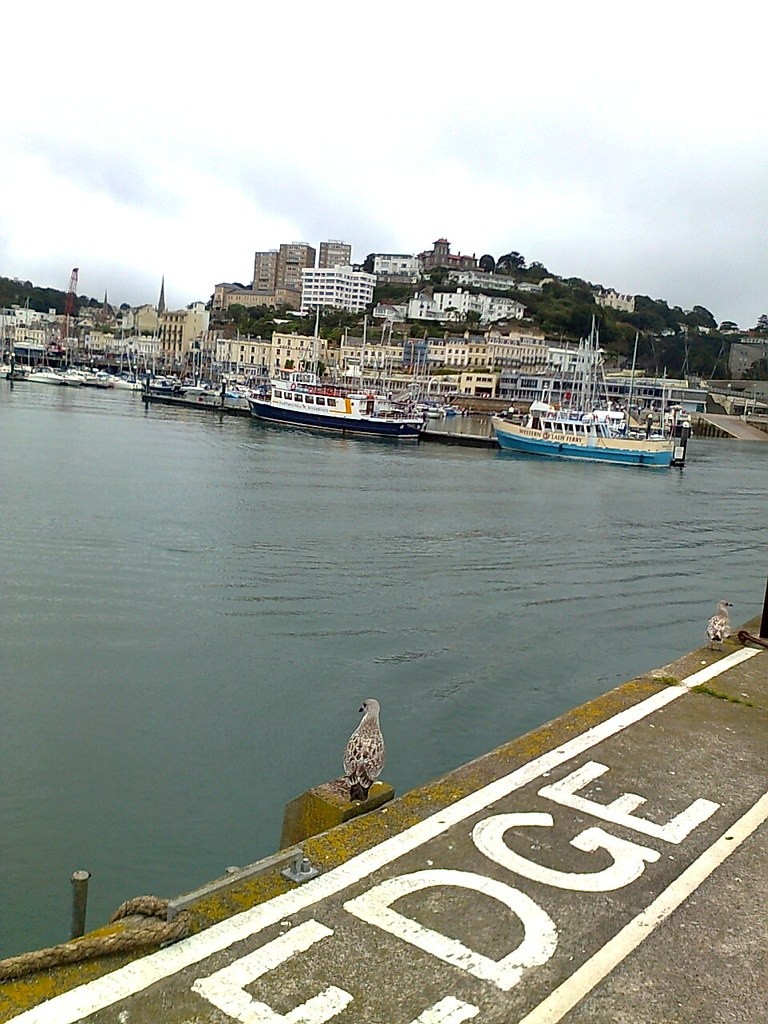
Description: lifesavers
xmin=291 ymin=383 xmax=296 ymax=391
xmin=543 ymin=432 xmax=550 ymax=439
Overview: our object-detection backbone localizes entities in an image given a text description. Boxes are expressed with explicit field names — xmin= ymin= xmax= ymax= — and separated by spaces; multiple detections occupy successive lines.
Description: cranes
xmin=60 ymin=267 xmax=79 ymax=340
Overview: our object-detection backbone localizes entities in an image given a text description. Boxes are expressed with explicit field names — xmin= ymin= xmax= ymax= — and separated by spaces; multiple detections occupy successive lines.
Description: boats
xmin=245 ymin=305 xmax=424 ymax=438
xmin=0 ymin=297 xmax=468 ymax=419
xmin=491 ymin=314 xmax=675 ymax=467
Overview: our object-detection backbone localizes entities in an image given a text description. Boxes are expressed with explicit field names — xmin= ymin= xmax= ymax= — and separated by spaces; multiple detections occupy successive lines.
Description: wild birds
xmin=706 ymin=600 xmax=733 ymax=651
xmin=343 ymin=698 xmax=385 ymax=803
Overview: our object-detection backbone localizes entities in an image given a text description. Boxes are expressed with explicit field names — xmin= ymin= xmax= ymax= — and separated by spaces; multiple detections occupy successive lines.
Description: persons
xmin=367 ymin=393 xmax=375 ymax=418
